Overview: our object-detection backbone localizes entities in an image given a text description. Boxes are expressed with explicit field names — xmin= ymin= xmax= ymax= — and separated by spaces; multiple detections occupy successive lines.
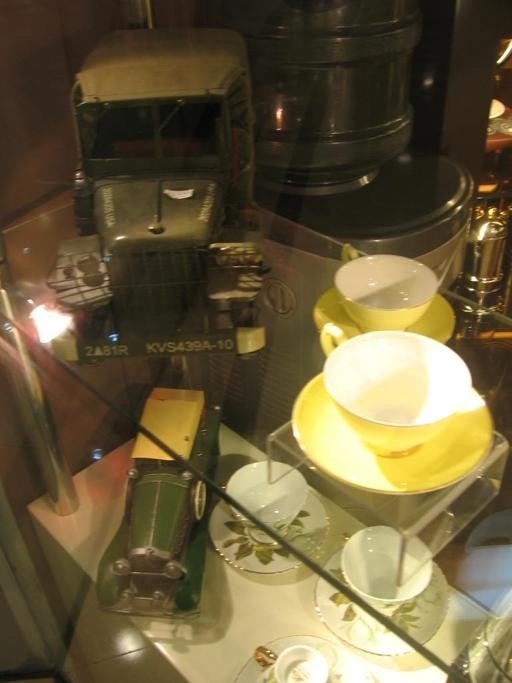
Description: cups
xmin=223 ymin=459 xmax=310 ymax=538
xmin=316 ymin=320 xmax=487 ymax=457
xmin=276 ymin=643 xmax=331 ymax=683
xmin=330 ymin=254 xmax=439 ymax=328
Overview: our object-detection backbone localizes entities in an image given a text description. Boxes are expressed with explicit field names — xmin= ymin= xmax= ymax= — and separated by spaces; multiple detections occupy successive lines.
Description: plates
xmin=226 ymin=634 xmax=376 ymax=683
xmin=310 ymin=287 xmax=460 ymax=347
xmin=290 ymin=277 xmax=496 ymax=499
xmin=291 ymin=373 xmax=496 ymax=498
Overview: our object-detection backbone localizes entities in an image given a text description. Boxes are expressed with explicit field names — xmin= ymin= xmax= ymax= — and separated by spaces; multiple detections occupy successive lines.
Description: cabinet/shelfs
xmin=2 ymin=169 xmax=512 ymax=680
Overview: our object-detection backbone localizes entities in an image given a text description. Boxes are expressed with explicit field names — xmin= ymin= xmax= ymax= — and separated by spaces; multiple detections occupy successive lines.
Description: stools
xmin=71 ymin=26 xmax=254 ymax=202
